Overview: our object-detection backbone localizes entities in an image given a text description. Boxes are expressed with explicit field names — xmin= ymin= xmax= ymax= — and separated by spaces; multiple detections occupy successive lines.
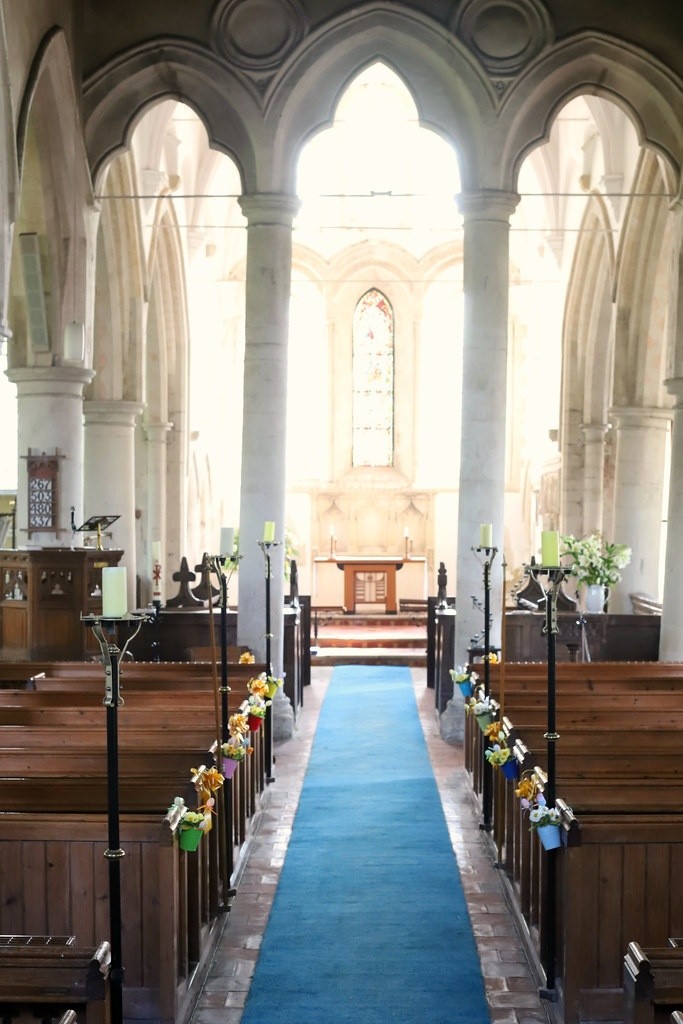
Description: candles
xmin=102 ymin=564 xmax=127 ymax=619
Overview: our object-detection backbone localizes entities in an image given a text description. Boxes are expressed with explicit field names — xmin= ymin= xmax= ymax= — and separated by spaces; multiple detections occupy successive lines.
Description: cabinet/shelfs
xmin=338 ymin=562 xmax=404 ymax=615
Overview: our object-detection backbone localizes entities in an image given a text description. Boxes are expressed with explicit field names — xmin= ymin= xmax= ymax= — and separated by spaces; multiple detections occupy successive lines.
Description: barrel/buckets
xmin=247 ymin=713 xmax=265 ymax=732
xmin=264 ymin=682 xmax=277 ymax=700
xmin=536 ymin=821 xmax=561 ymax=851
xmin=221 ymin=757 xmax=239 ymax=780
xmin=476 ymin=712 xmax=493 ymax=732
xmin=500 ymin=756 xmax=518 ymax=779
xmin=177 ymin=826 xmax=205 ymax=853
xmin=460 ymin=679 xmax=474 ymax=698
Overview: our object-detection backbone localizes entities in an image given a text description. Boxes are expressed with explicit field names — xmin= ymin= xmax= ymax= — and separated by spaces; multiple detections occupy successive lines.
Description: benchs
xmin=464 ymin=662 xmax=683 ymax=1024
xmin=0 ymin=662 xmax=277 ymax=1024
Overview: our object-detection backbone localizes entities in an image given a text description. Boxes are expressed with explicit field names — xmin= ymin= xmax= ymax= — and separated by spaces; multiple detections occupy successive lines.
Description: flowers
xmin=560 ymin=531 xmax=632 ymax=585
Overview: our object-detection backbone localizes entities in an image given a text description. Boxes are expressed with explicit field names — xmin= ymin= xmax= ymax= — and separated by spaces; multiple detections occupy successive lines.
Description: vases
xmin=581 ymin=585 xmax=611 ymax=614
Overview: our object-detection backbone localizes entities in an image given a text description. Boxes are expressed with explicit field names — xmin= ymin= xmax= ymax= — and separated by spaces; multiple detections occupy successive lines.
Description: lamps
xmin=480 ymin=522 xmax=492 ymax=547
xmin=264 ymin=521 xmax=275 ymax=540
xmin=220 ymin=526 xmax=234 ymax=555
xmin=540 ymin=529 xmax=561 ymax=567
